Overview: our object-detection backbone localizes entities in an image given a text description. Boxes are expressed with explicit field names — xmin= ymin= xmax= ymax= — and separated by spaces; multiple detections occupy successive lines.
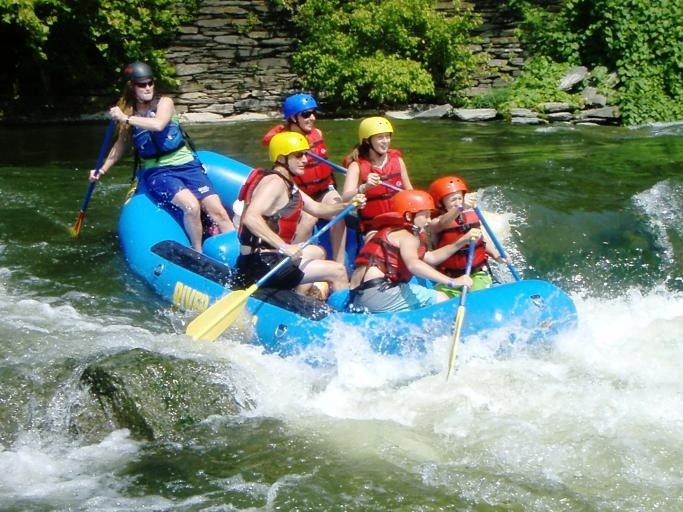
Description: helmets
xmin=359 ymin=117 xmax=393 ymax=145
xmin=269 ymin=131 xmax=309 ymax=163
xmin=123 ymin=62 xmax=152 ymax=81
xmin=429 ymin=176 xmax=467 ymax=205
xmin=283 ymin=94 xmax=317 ymax=117
xmin=395 ymin=190 xmax=434 ymax=216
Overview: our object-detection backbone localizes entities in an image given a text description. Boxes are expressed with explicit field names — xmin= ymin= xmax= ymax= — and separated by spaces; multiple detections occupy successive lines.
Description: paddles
xmin=186 ymin=203 xmax=358 ymax=343
xmin=72 ymin=116 xmax=116 ymax=237
xmin=445 ymin=236 xmax=475 ymax=386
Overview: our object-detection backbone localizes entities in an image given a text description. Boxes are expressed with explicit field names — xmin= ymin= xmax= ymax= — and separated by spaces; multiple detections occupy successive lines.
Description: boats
xmin=118 ymin=151 xmax=577 ymax=385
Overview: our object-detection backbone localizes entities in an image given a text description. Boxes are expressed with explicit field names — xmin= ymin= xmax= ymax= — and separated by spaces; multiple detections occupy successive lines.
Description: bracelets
xmin=99 ymin=166 xmax=108 ymax=176
xmin=126 ymin=113 xmax=132 ymax=124
xmin=445 ymin=276 xmax=455 ymax=287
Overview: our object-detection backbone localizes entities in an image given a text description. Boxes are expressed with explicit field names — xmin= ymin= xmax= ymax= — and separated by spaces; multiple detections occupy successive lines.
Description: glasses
xmin=292 ymin=151 xmax=309 ymax=158
xmin=301 ymin=111 xmax=316 ymax=117
xmin=134 ymin=81 xmax=153 ymax=87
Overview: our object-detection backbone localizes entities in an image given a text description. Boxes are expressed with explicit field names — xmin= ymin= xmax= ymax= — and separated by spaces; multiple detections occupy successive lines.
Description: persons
xmin=236 ymin=129 xmax=367 ymax=294
xmin=344 ymin=117 xmax=413 ymax=204
xmin=90 ymin=59 xmax=239 ymax=254
xmin=427 ymin=176 xmax=514 ymax=286
xmin=345 ymin=190 xmax=473 ymax=310
xmin=262 ymin=93 xmax=345 ymax=264
